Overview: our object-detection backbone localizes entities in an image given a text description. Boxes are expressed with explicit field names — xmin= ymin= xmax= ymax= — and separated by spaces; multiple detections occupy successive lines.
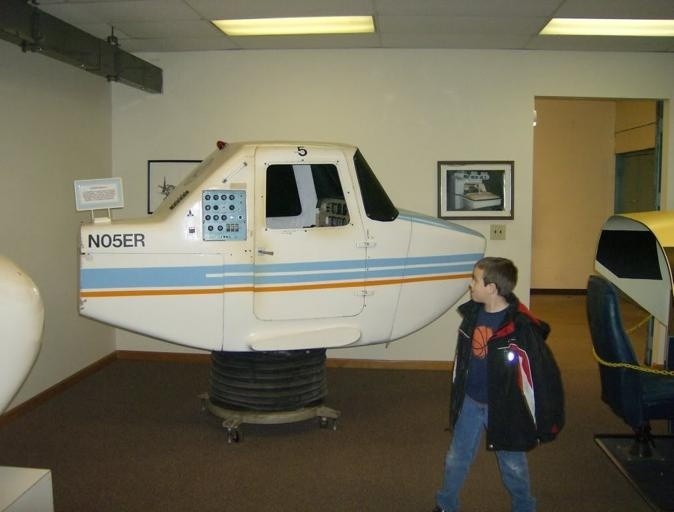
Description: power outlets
xmin=490 ymin=223 xmax=507 ymax=240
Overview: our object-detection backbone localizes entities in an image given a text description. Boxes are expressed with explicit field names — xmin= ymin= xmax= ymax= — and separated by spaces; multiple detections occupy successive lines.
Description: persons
xmin=430 ymin=256 xmax=551 ymax=512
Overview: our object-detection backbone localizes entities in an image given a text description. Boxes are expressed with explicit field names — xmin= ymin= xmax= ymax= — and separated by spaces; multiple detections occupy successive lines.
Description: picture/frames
xmin=438 ymin=161 xmax=514 ymax=220
xmin=148 ymin=160 xmax=203 ymax=214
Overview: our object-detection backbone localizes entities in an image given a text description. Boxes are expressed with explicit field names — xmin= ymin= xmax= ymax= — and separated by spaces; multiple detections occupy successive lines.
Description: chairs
xmin=587 ymin=276 xmax=674 ymax=463
